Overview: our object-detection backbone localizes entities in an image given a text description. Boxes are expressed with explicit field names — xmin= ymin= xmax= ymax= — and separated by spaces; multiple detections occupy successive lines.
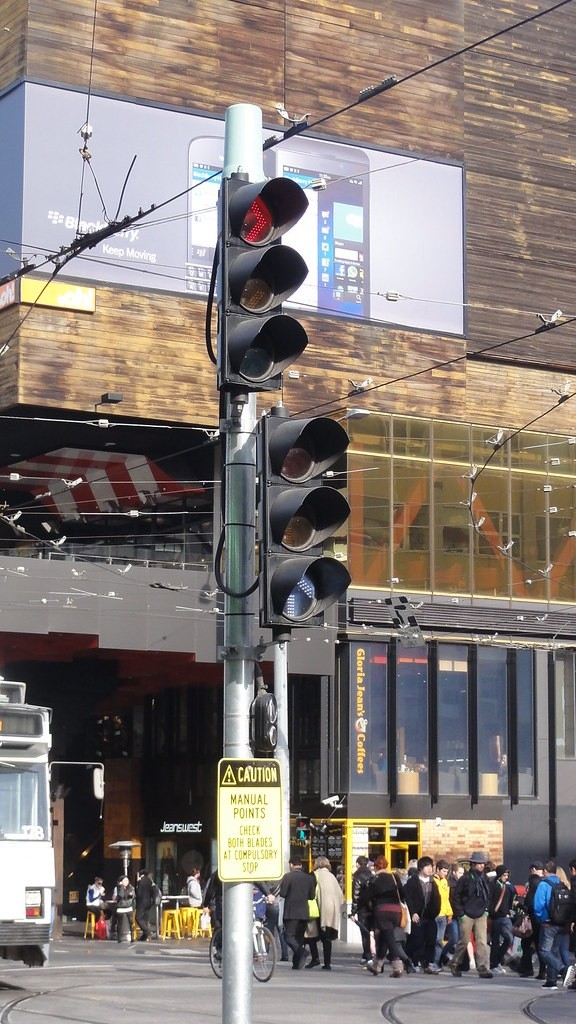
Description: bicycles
xmin=208 ymin=894 xmax=279 ymax=982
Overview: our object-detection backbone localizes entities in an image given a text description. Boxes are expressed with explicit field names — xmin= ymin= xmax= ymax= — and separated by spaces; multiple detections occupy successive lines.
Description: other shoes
xmin=280 ymin=947 xmax=331 ymax=971
xmin=406 ymin=957 xmax=413 ymax=974
xmin=380 ymin=964 xmax=384 ymax=973
xmin=491 ymin=963 xmax=506 ymax=976
xmin=360 ymin=957 xmax=374 ymax=968
xmin=520 ymin=971 xmax=534 ymax=977
xmin=479 ymin=970 xmax=493 ymax=978
xmin=535 ymin=974 xmax=546 ymax=980
xmin=412 ymin=956 xmax=470 ymax=974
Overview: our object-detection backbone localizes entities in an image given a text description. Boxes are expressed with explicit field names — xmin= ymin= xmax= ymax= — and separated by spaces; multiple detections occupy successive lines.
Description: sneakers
xmin=561 ymin=965 xmax=575 ymax=988
xmin=541 ymin=981 xmax=558 ymax=990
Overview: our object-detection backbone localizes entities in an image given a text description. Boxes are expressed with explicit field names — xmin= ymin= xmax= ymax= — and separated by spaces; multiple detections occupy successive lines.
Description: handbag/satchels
xmin=486 ymin=915 xmax=493 ymax=934
xmin=401 ymin=902 xmax=412 ymax=935
xmin=308 ymin=899 xmax=320 ymax=920
xmin=511 ymin=905 xmax=532 ymax=938
xmin=399 ymin=907 xmax=408 ymax=929
xmin=201 ymin=912 xmax=211 ymax=930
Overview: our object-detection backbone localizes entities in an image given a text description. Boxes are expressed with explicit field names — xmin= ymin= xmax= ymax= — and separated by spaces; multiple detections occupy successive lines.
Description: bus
xmin=0 ymin=676 xmax=108 ymax=966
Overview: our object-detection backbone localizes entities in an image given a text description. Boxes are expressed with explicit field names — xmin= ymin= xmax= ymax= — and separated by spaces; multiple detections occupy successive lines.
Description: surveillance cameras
xmin=322 ymin=795 xmax=339 ymax=805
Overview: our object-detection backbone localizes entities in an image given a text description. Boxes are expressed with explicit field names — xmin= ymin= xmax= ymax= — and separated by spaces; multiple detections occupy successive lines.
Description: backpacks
xmin=540 ymin=879 xmax=573 ymax=928
xmin=179 ymin=879 xmax=196 ymax=907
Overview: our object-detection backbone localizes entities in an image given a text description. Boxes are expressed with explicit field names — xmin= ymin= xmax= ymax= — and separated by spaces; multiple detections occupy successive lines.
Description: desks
xmin=376 ymin=770 xmax=419 ymax=795
xmin=458 ymin=773 xmax=497 ymax=796
xmin=155 ymin=895 xmax=189 ymax=939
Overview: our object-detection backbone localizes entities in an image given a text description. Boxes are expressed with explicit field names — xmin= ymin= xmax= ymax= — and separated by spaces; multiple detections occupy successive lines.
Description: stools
xmin=131 ymin=917 xmax=144 ymax=942
xmin=159 ymin=907 xmax=212 ymax=941
xmin=82 ymin=909 xmax=104 ymax=940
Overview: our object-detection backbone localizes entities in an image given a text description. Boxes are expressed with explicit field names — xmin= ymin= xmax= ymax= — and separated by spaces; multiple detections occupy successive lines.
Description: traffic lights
xmin=295 ymin=816 xmax=312 ymax=840
xmin=219 ymin=176 xmax=310 ymax=392
xmin=257 ymin=413 xmax=355 ymax=629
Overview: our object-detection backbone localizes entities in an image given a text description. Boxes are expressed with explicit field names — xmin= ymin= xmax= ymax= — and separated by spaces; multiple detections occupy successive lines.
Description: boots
xmin=366 ymin=959 xmax=384 ymax=976
xmin=390 ymin=960 xmax=403 ymax=978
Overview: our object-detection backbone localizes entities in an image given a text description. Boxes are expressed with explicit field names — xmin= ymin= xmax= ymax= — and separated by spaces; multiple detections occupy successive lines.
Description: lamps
xmin=95 ymin=393 xmax=123 ymax=407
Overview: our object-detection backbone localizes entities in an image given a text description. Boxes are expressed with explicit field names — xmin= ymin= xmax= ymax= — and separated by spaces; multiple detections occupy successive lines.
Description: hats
xmin=467 ymin=852 xmax=487 ymax=863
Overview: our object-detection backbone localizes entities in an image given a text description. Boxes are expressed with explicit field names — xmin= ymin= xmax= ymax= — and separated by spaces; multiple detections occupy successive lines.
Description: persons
xmin=252 ymin=855 xmax=343 ymax=970
xmin=352 ymin=852 xmax=576 ymax=990
xmin=87 ymin=865 xmax=223 ymax=959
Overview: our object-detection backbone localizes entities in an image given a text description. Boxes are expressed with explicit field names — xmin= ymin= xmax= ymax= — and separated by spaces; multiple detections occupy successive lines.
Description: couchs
xmin=420 ymin=766 xmax=456 ymax=793
xmin=518 ymin=768 xmax=534 ymax=796
